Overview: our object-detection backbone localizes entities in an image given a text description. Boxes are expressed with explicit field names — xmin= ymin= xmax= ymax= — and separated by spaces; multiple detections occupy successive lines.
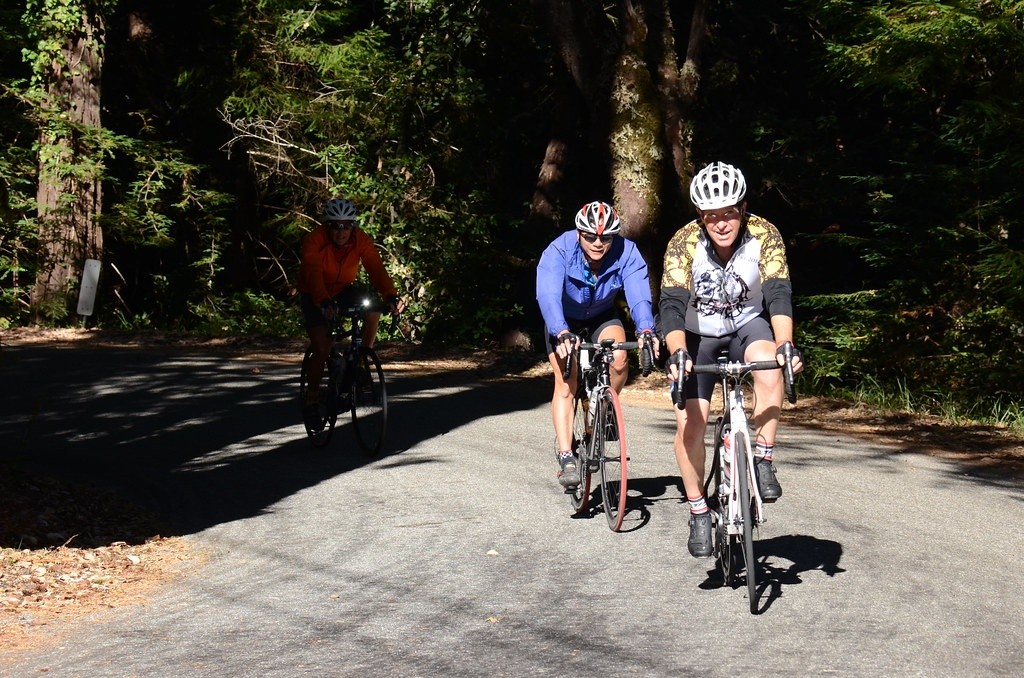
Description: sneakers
xmin=606 ymin=402 xmax=618 ymax=441
xmin=753 ymin=455 xmax=783 ymax=499
xmin=557 ymin=456 xmax=581 ymax=486
xmin=688 ymin=511 xmax=713 ymax=559
xmin=356 ymin=359 xmax=373 ymax=384
xmin=302 ymin=403 xmax=324 ymax=431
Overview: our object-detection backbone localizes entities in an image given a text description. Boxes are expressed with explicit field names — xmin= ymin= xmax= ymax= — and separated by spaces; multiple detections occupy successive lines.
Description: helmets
xmin=574 ymin=200 xmax=622 ymax=235
xmin=689 ymin=160 xmax=747 ymax=211
xmin=322 ymin=198 xmax=358 ymax=222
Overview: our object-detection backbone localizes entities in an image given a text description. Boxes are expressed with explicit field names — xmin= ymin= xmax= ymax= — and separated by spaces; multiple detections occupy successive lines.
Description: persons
xmin=668 ymin=162 xmax=808 ymax=557
xmin=537 ymin=199 xmax=664 ymax=491
xmin=295 ymin=198 xmax=401 ymax=434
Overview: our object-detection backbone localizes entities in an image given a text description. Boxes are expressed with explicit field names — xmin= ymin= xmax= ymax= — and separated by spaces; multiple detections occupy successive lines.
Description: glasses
xmin=579 ymin=231 xmax=614 ymax=244
xmin=696 ymin=207 xmax=744 ymax=221
xmin=328 ymin=223 xmax=354 ymax=230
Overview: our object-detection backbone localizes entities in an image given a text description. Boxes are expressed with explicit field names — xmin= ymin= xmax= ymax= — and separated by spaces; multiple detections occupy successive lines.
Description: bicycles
xmin=672 ymin=340 xmax=798 ymax=615
xmin=561 ymin=332 xmax=654 ymax=531
xmin=299 ymin=294 xmax=399 ymax=455
